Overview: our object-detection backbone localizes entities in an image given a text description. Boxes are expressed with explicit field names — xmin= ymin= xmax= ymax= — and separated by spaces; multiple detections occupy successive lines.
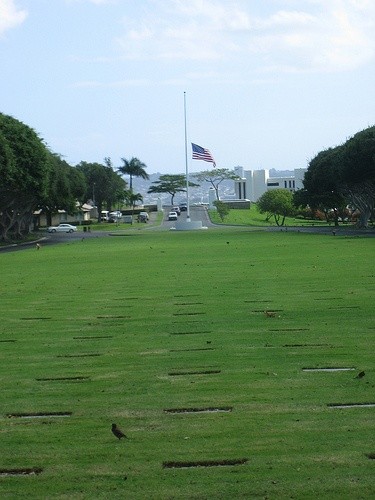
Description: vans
xmin=100 ymin=210 xmax=122 ymax=223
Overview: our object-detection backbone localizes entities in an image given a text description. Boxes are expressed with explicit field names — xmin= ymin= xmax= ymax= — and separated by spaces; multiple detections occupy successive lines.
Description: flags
xmin=191 ymin=142 xmax=216 ymax=168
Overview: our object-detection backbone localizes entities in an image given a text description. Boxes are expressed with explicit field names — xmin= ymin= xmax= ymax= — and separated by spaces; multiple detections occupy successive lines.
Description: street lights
xmin=92 ymin=182 xmax=96 ymax=204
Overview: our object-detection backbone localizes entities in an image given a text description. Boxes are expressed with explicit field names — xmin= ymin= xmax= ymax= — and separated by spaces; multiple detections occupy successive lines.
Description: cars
xmin=138 ymin=212 xmax=149 ymax=223
xmin=172 ymin=207 xmax=181 ymax=216
xmin=48 ymin=224 xmax=77 ymax=233
xmin=168 ymin=211 xmax=178 ymax=220
xmin=179 ymin=202 xmax=187 ymax=212
